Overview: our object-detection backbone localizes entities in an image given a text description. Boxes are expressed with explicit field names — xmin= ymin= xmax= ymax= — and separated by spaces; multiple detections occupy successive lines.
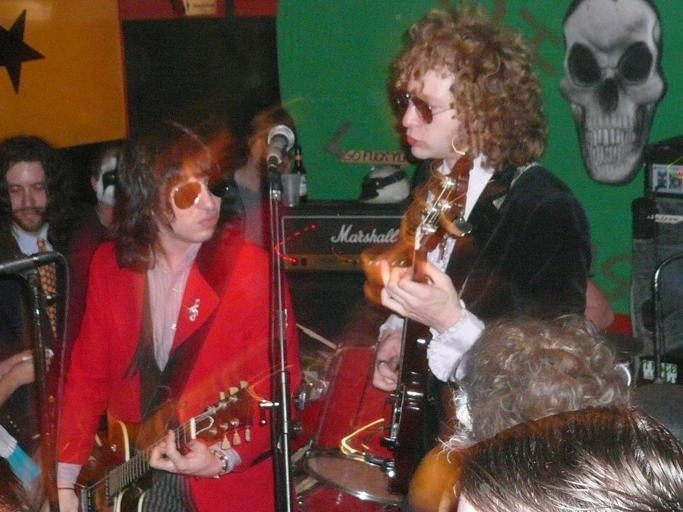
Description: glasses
xmin=392 ymin=85 xmax=459 ymax=126
xmin=159 ymin=170 xmax=229 ymax=209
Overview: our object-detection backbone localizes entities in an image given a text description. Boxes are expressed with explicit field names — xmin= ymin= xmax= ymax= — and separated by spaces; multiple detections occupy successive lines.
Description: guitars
xmin=74 ymin=380 xmax=261 ymax=511
xmin=379 ymin=169 xmax=475 ymax=495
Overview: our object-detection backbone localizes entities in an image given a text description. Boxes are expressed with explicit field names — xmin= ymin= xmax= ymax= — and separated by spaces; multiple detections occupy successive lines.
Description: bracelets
xmin=57 ymin=488 xmax=76 ymax=490
xmin=210 ymin=450 xmax=229 ymax=479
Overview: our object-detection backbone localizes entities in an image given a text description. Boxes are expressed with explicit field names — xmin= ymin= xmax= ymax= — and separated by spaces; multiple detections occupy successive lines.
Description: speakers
xmin=279 ymin=199 xmax=408 ymax=361
xmin=629 ymin=198 xmax=683 ymax=385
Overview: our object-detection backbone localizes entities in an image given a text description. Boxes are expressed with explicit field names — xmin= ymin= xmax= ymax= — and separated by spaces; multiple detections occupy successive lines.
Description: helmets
xmin=358 ymin=163 xmax=413 ymax=206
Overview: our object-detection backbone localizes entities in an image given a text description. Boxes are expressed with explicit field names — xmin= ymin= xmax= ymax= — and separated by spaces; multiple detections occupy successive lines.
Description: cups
xmin=281 ymin=173 xmax=302 ymax=209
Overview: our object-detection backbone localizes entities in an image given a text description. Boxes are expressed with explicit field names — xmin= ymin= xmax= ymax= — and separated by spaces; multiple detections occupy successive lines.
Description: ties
xmin=35 ymin=236 xmax=59 ymax=343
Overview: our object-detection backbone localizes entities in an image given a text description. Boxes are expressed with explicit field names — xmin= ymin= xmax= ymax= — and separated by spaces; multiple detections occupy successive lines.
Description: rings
xmin=377 ymin=360 xmax=388 ymax=370
xmin=389 ymin=294 xmax=395 ymax=299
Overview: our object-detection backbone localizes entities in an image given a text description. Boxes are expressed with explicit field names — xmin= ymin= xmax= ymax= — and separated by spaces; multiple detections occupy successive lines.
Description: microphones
xmin=0 ymin=252 xmax=64 ymax=281
xmin=265 ymin=124 xmax=295 ymax=177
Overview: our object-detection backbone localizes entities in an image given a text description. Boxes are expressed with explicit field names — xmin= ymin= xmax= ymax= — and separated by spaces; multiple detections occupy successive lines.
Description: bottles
xmin=291 ymin=144 xmax=309 ymax=204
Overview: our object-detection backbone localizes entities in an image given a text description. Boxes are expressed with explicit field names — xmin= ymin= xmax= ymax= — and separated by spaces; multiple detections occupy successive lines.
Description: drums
xmin=289 ymin=371 xmax=330 ymax=503
xmin=302 ymin=340 xmax=410 ymax=506
xmin=298 ymin=483 xmax=400 ymax=511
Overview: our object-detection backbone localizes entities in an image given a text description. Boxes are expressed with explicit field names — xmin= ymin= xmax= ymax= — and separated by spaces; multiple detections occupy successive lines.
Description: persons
xmin=42 ymin=114 xmax=302 ymax=512
xmin=443 ymin=315 xmax=633 ymax=462
xmin=218 ymin=105 xmax=296 ymax=245
xmin=463 ymin=406 xmax=682 ymax=510
xmin=585 ymin=282 xmax=617 ymax=327
xmin=1 ymin=135 xmax=118 ymax=402
xmin=358 ymin=11 xmax=589 ymax=394
